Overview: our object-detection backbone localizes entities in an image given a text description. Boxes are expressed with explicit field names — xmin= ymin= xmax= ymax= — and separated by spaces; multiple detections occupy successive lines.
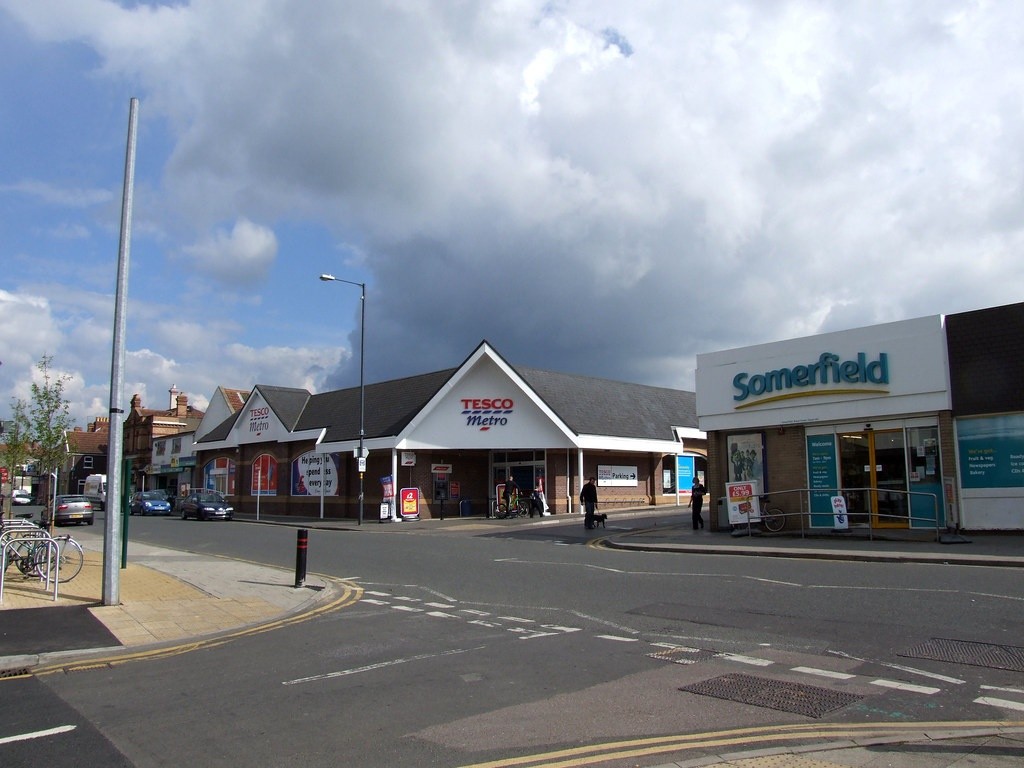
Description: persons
xmin=503 ymin=475 xmax=523 ymax=519
xmin=687 ymin=476 xmax=707 ymax=529
xmin=580 ymin=477 xmax=599 ymax=529
xmin=529 ymin=483 xmax=545 ymax=518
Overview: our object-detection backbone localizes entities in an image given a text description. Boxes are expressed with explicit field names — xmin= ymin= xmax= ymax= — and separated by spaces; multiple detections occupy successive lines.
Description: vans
xmin=83 ymin=474 xmax=107 ymax=510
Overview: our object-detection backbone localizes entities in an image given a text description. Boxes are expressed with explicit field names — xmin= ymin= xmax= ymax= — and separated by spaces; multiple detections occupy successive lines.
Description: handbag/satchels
xmin=694 ymin=485 xmax=708 ymax=495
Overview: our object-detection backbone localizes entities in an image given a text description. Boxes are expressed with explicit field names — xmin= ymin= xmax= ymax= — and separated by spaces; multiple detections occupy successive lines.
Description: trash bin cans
xmin=461 ymin=500 xmax=472 ymax=517
xmin=716 ymin=496 xmax=732 ymax=533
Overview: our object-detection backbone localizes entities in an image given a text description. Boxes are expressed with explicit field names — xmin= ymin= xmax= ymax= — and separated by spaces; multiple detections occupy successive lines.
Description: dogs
xmin=593 ymin=514 xmax=607 ymax=528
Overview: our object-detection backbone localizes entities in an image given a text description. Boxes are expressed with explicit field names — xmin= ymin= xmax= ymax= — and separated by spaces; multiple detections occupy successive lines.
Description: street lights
xmin=320 ymin=274 xmax=366 ymax=526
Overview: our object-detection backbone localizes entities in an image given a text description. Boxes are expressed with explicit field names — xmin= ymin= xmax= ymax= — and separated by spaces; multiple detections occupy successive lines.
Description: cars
xmin=12 ymin=493 xmax=32 ymax=505
xmin=179 ymin=492 xmax=234 ymax=521
xmin=41 ymin=495 xmax=94 ymax=525
xmin=128 ymin=488 xmax=175 ymax=516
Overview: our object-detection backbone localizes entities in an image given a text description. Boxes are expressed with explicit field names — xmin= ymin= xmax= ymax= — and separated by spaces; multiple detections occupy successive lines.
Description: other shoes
xmin=530 ymin=516 xmax=533 ymax=518
xmin=591 ymin=526 xmax=595 ymax=529
xmin=584 ymin=526 xmax=589 ymax=529
xmin=540 ymin=515 xmax=545 ymax=517
xmin=693 ymin=527 xmax=699 ymax=530
xmin=701 ymin=523 xmax=704 ymax=528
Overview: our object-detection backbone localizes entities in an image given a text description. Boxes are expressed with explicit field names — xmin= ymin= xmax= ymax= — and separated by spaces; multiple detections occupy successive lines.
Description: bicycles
xmin=760 ymin=499 xmax=786 ymax=532
xmin=0 ymin=512 xmax=84 ymax=584
xmin=494 ymin=493 xmax=529 ymax=519
